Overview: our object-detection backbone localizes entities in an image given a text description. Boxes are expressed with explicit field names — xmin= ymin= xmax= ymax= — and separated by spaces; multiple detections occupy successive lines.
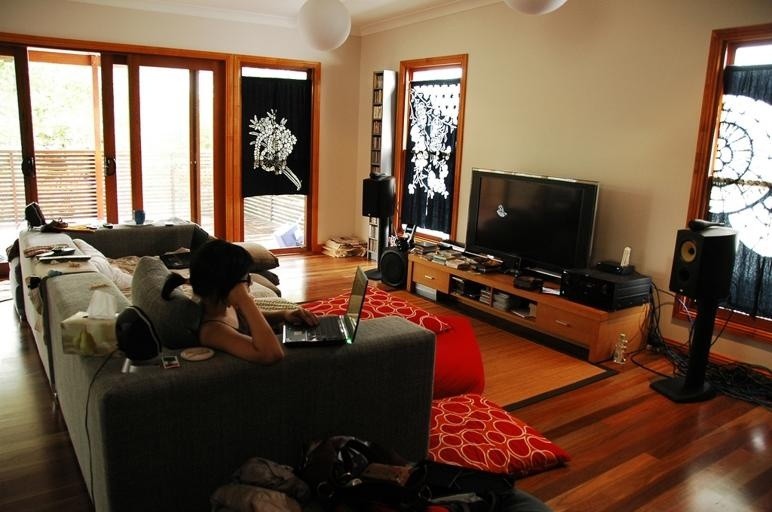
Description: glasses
xmin=242 ymin=275 xmax=253 ymax=285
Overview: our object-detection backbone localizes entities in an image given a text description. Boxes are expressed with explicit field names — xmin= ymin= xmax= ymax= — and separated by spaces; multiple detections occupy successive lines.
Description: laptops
xmin=280 ymin=265 xmax=370 ymax=345
xmin=159 ymin=223 xmax=210 ymax=270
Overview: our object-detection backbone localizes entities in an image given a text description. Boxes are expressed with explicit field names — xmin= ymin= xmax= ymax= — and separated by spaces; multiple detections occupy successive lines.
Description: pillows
xmin=132 ymin=255 xmax=202 ymax=348
xmin=298 ymin=286 xmax=451 ymax=333
xmin=433 ymin=316 xmax=485 ymax=396
xmin=73 ymin=239 xmax=133 ymax=288
xmin=428 ymin=395 xmax=572 ymax=476
xmin=230 ymin=241 xmax=278 ymax=271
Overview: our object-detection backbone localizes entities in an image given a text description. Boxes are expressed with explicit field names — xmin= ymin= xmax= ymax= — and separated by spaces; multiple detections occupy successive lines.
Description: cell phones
xmin=103 ymin=224 xmax=113 ymax=229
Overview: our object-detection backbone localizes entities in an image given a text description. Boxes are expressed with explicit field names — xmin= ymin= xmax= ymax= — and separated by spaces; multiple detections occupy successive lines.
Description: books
xmin=412 ymin=241 xmax=469 ymax=270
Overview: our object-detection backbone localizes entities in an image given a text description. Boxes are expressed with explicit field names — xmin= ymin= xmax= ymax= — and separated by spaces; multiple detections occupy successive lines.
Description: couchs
xmin=6 ymin=217 xmax=436 ymax=512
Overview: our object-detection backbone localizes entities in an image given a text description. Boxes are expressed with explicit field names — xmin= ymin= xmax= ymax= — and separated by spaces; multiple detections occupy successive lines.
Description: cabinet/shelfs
xmin=407 ymin=238 xmax=650 ymax=364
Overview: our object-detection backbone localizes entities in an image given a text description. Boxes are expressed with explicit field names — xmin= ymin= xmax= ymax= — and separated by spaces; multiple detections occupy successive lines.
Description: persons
xmin=159 ymin=239 xmax=327 ymax=365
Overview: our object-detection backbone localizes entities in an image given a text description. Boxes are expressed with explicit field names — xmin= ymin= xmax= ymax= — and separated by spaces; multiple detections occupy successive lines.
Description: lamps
xmin=297 ymin=1 xmax=351 ymax=53
xmin=502 ymin=0 xmax=567 ymax=16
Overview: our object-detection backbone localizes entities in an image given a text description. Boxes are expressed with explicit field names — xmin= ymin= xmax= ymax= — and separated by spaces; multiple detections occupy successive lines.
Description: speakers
xmin=24 ymin=201 xmax=46 ymax=232
xmin=115 ymin=305 xmax=164 ymax=374
xmin=667 ymin=226 xmax=738 ymax=303
xmin=378 ymin=245 xmax=417 ymax=290
xmin=362 ymin=175 xmax=398 ymax=219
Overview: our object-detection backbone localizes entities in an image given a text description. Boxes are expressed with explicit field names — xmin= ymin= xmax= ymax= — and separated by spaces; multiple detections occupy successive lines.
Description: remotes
xmin=36 ymin=247 xmax=76 ymax=260
xmin=38 ymin=255 xmax=92 ymax=261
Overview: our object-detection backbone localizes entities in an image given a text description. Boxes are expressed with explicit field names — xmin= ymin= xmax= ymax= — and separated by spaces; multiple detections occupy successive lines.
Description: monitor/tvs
xmin=464 ymin=166 xmax=600 ymax=277
